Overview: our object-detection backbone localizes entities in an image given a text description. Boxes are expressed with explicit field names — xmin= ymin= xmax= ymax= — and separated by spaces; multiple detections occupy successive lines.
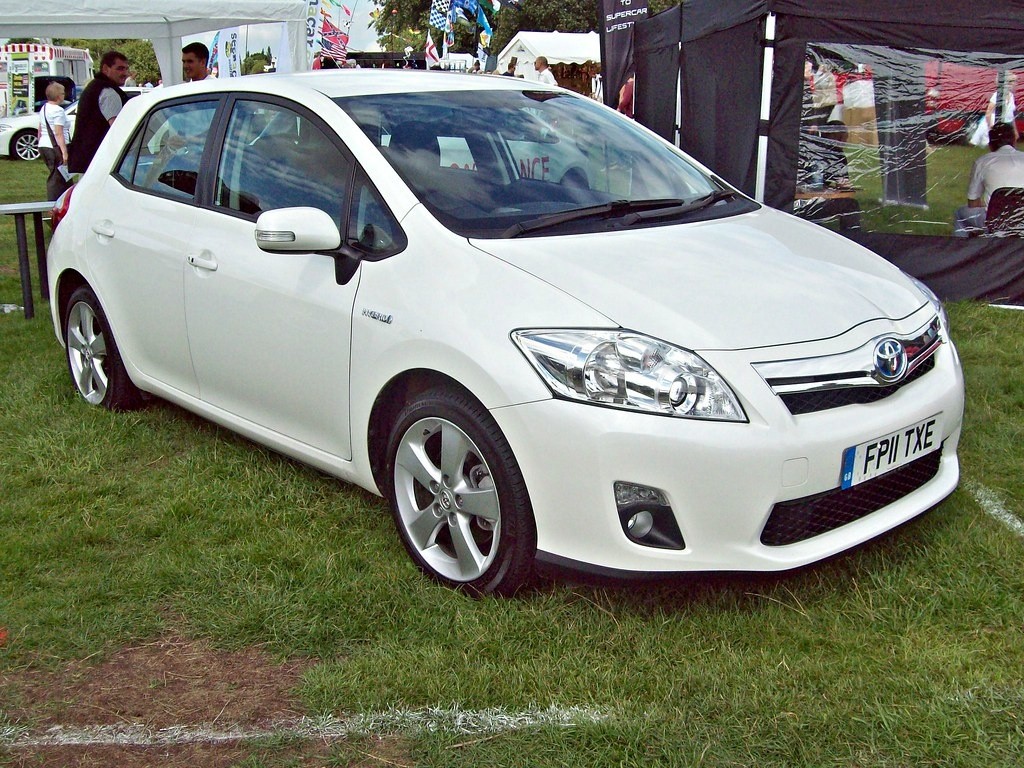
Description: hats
xmin=346 ymin=59 xmax=357 ymax=66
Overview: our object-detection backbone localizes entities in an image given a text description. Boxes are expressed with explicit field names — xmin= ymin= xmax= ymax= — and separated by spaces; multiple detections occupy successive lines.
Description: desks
xmin=0 ymin=201 xmax=56 ymax=319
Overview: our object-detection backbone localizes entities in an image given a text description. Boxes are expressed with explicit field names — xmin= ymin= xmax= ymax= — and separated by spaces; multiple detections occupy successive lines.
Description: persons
xmin=616 ymin=73 xmax=634 ymax=118
xmin=534 ymin=56 xmax=558 ymax=86
xmin=311 ymin=52 xmax=361 ymax=70
xmin=38 ymin=82 xmax=71 ymax=201
xmin=124 ymin=70 xmax=162 ymax=88
xmin=969 ymin=71 xmax=1021 ymax=150
xmin=67 ymin=51 xmax=130 ymax=181
xmin=271 ymin=55 xmax=276 ymax=68
xmin=953 ymin=122 xmax=1024 ymax=237
xmin=795 ymin=42 xmax=851 ymax=194
xmin=468 ymin=60 xmax=515 ymax=76
xmin=182 ymin=42 xmax=217 ymax=83
xmin=547 ymin=60 xmax=603 ymax=103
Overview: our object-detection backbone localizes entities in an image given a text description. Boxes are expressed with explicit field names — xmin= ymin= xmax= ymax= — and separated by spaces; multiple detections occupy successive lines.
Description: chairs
xmin=389 ymin=120 xmax=481 ymax=221
xmin=246 ymin=132 xmax=342 ymax=229
xmin=985 ymin=187 xmax=1024 ymax=238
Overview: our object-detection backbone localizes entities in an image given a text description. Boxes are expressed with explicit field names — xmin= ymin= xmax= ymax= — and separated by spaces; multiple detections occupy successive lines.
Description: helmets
xmin=390 ymin=121 xmax=442 ymax=166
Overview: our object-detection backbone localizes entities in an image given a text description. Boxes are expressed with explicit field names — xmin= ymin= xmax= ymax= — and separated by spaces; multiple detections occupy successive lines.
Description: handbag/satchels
xmin=54 ymin=142 xmax=72 ymax=165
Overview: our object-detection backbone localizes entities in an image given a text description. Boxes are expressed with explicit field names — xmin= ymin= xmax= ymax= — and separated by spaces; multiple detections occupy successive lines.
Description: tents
xmin=0 ymin=0 xmax=309 ymax=88
xmin=599 ymin=0 xmax=1024 ymax=309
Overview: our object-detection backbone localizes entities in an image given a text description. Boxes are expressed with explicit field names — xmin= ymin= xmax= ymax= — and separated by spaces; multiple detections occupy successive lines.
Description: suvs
xmin=0 ymin=86 xmax=163 ymax=161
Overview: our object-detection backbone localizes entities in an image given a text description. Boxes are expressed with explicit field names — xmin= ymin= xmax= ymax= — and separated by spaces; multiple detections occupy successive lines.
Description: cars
xmin=42 ymin=66 xmax=966 ymax=603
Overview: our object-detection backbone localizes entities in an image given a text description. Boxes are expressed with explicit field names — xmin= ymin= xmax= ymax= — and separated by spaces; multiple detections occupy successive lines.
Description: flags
xmin=315 ymin=0 xmax=354 ymax=61
xmin=424 ymin=0 xmax=521 ymax=73
